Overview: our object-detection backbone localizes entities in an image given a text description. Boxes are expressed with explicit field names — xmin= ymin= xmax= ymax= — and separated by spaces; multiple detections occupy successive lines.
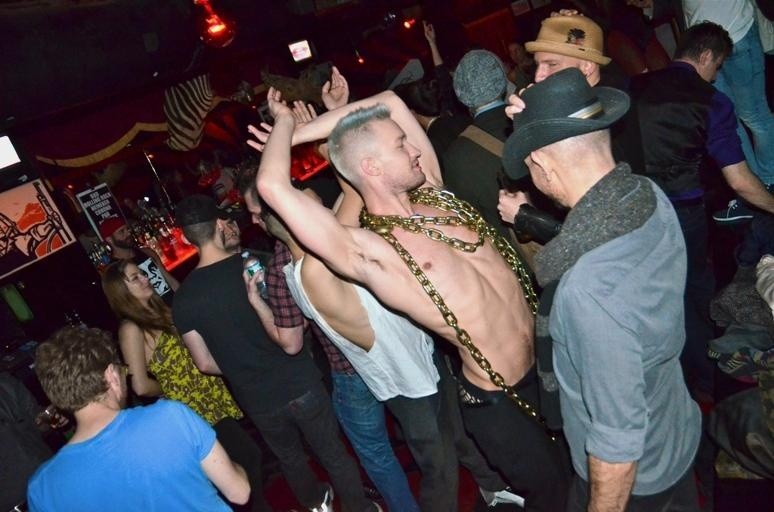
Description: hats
xmin=525 ymin=15 xmax=611 ymax=65
xmin=453 ymin=50 xmax=508 ymax=107
xmin=171 ymin=199 xmax=229 ymax=229
xmin=504 ymin=67 xmax=631 ymax=180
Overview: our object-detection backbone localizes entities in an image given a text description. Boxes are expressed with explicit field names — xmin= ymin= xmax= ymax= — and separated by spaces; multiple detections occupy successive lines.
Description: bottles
xmin=129 ymin=207 xmax=192 ymax=264
xmin=88 ymin=242 xmax=113 ymax=265
xmin=241 ymin=251 xmax=268 ymax=300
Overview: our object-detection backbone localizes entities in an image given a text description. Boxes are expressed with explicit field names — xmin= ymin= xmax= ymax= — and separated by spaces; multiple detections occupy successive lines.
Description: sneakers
xmin=713 ymin=199 xmax=755 ymax=221
xmin=311 ymin=485 xmax=334 ymax=512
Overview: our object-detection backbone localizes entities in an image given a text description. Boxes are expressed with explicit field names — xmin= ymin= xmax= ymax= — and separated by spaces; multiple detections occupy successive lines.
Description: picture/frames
xmin=0 ymin=174 xmax=80 ymax=286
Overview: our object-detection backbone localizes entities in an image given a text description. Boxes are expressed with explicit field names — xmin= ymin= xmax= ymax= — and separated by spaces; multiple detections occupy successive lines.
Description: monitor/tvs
xmin=288 ymin=39 xmax=312 ymax=63
xmin=0 ymin=135 xmax=21 ymax=169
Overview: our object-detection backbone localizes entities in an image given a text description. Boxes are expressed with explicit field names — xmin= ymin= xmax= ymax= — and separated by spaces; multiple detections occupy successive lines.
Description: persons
xmin=0 ymin=0 xmax=774 ymax=512
